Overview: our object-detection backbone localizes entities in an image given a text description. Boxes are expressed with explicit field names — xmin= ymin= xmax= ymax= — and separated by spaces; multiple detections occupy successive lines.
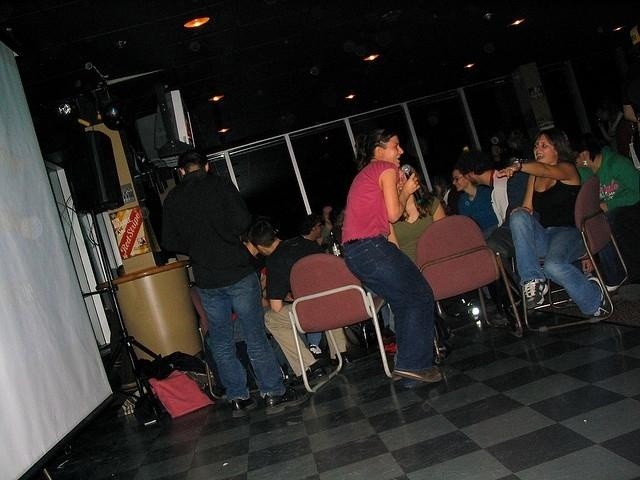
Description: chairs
xmin=521 ymin=174 xmax=629 ymax=335
xmin=191 ymin=286 xmax=262 ymax=401
xmin=412 ymin=213 xmax=523 ymax=370
xmin=287 ymin=250 xmax=395 ymax=397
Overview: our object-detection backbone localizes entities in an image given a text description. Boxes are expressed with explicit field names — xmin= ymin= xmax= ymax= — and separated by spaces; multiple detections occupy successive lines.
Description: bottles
xmin=328 ymin=231 xmax=342 ymax=259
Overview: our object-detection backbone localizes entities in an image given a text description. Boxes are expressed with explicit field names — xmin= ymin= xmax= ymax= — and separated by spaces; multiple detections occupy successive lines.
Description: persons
xmin=622 ymin=26 xmax=639 ymax=158
xmin=250 ymin=204 xmax=397 ymax=396
xmin=590 ymin=98 xmax=623 ymax=145
xmin=385 ymin=130 xmax=639 ymax=352
xmin=160 ymin=147 xmax=310 ymax=416
xmin=342 ymin=130 xmax=444 ymax=388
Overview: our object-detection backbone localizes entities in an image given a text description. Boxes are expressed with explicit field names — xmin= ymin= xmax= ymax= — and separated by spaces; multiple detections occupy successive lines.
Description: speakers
xmin=67 ymin=129 xmax=125 ymax=213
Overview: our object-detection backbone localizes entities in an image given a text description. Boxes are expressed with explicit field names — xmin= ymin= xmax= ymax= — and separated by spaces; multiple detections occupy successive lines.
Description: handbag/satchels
xmin=148 ymin=370 xmax=214 ymax=418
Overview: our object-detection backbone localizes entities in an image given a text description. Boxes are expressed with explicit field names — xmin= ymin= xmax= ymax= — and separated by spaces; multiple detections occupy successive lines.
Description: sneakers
xmin=523 ymin=279 xmax=548 ymax=309
xmin=587 ymin=277 xmax=611 ymax=319
xmin=391 ymin=368 xmax=443 ymax=382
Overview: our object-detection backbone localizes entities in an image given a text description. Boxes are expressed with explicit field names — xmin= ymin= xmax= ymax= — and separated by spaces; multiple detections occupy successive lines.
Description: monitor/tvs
xmin=136 ymin=90 xmax=198 ymax=157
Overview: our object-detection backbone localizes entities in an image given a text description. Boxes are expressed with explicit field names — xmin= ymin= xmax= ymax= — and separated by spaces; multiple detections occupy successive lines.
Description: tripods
xmin=86 ymin=222 xmax=173 ymax=414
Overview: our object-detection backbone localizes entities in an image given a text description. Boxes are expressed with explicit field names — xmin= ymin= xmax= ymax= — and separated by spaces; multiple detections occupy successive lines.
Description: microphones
xmin=400 ymin=164 xmax=423 ymax=204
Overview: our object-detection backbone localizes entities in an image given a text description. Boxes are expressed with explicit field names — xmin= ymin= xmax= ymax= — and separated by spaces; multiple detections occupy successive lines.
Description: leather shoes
xmin=231 ymin=396 xmax=257 ymax=417
xmin=266 ymin=388 xmax=310 ymax=414
xmin=606 ymin=276 xmax=627 ymax=291
xmin=293 ymin=368 xmax=328 ymax=390
